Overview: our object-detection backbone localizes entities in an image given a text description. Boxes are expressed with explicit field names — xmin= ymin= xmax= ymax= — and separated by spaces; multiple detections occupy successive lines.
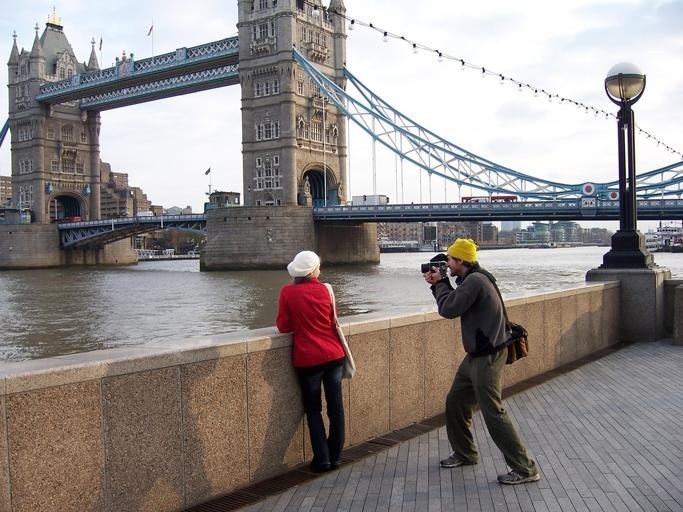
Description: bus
xmin=460 ymin=196 xmax=519 ymax=203
xmin=49 ymin=216 xmax=80 ymax=224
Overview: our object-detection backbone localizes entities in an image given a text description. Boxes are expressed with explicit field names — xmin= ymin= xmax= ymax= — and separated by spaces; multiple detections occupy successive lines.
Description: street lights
xmin=592 ymin=62 xmax=655 ymax=269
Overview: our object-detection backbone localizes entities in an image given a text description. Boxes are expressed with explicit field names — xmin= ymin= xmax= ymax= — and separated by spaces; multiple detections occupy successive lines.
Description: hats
xmin=447 ymin=238 xmax=478 ymax=263
xmin=286 ymin=250 xmax=319 ymax=278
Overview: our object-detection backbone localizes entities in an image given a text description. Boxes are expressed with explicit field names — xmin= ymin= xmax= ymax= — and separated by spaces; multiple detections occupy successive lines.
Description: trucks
xmin=344 ymin=194 xmax=389 ymax=212
xmin=135 ymin=211 xmax=154 ymax=221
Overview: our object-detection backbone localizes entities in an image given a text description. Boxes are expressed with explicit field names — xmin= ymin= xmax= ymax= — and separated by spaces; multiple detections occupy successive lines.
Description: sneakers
xmin=439 ymin=456 xmax=476 ymax=467
xmin=302 ymin=462 xmax=340 ymax=475
xmin=496 ymin=470 xmax=540 ymax=485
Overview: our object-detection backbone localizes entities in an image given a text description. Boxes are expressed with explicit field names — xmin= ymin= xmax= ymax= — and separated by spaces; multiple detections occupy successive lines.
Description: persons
xmin=423 ymin=238 xmax=540 ymax=484
xmin=276 ymin=250 xmax=347 ymax=473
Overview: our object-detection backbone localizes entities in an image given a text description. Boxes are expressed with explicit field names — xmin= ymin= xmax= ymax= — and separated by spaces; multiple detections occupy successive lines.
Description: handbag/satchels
xmin=505 ymin=320 xmax=529 ymax=364
xmin=335 ymin=322 xmax=357 ymax=380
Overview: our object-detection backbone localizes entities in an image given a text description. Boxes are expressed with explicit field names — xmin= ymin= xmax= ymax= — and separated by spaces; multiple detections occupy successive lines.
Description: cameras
xmin=420 ymin=261 xmax=448 ymax=278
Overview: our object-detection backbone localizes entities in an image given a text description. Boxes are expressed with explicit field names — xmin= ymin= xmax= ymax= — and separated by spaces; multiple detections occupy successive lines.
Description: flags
xmin=99 ymin=37 xmax=102 ymax=50
xmin=205 ymin=168 xmax=211 ymax=175
xmin=147 ymin=25 xmax=153 ymax=36
